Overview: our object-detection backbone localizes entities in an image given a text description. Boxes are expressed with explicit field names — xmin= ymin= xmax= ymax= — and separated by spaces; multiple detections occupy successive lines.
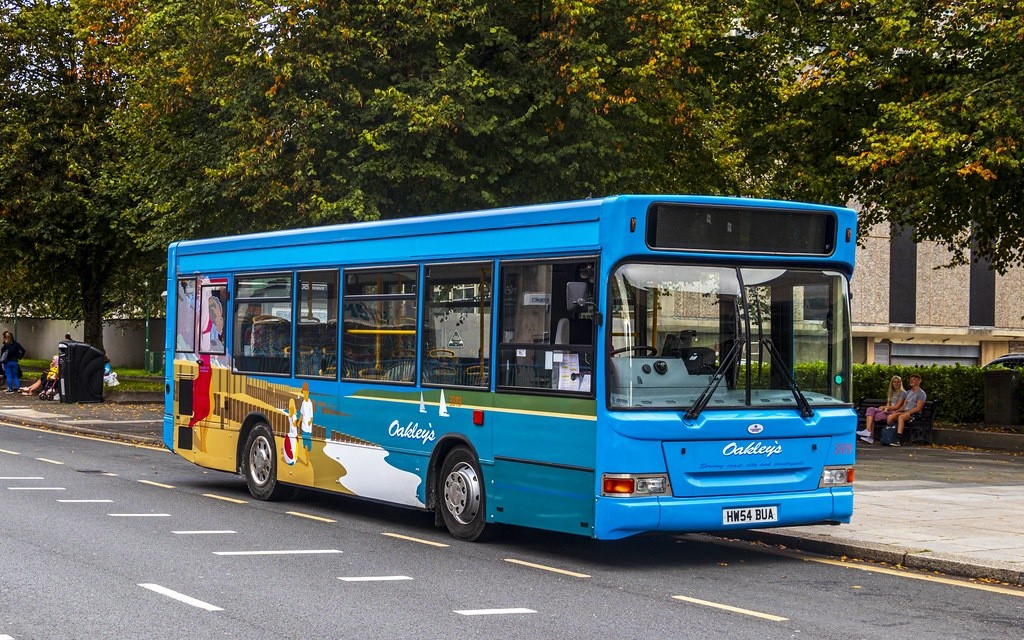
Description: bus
xmin=163 ymin=193 xmax=857 ymax=547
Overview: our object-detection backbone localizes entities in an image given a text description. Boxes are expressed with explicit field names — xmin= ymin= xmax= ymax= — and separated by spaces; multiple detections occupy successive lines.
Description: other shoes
xmin=856 ymin=429 xmax=871 ymax=436
xmin=22 ymin=391 xmax=32 ymax=396
xmin=19 ymin=387 xmax=29 ymax=391
xmin=4 ymin=391 xmax=14 ymax=395
xmin=860 ymin=436 xmax=874 ymax=444
xmin=891 ymin=441 xmax=901 ymax=447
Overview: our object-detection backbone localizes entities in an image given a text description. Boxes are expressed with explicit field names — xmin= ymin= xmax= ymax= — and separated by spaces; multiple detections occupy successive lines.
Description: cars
xmin=977 ymin=352 xmax=1024 ymax=371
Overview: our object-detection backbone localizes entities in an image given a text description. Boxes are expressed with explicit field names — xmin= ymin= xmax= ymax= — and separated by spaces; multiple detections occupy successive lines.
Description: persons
xmin=0 ymin=331 xmax=61 ymax=398
xmin=887 ymin=374 xmax=927 ymax=446
xmin=856 ymin=375 xmax=907 ymax=444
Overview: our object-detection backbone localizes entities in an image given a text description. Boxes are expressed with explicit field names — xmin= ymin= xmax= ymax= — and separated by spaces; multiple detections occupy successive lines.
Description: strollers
xmin=39 ymin=370 xmax=61 ymax=401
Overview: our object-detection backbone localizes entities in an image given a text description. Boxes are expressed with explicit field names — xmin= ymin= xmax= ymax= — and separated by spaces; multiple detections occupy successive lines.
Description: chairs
xmin=552 ymin=282 xmax=590 ymax=389
xmin=243 ymin=315 xmax=490 ymax=385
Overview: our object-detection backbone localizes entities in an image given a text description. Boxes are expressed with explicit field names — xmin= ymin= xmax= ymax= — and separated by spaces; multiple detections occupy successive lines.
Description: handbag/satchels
xmin=0 ymin=349 xmax=9 ymax=362
xmin=881 ymin=425 xmax=896 ymax=445
xmin=17 ymin=364 xmax=22 ymax=379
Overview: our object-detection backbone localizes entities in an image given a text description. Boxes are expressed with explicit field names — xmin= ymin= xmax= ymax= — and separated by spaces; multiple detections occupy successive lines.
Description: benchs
xmin=854 ymin=397 xmax=938 ymax=446
